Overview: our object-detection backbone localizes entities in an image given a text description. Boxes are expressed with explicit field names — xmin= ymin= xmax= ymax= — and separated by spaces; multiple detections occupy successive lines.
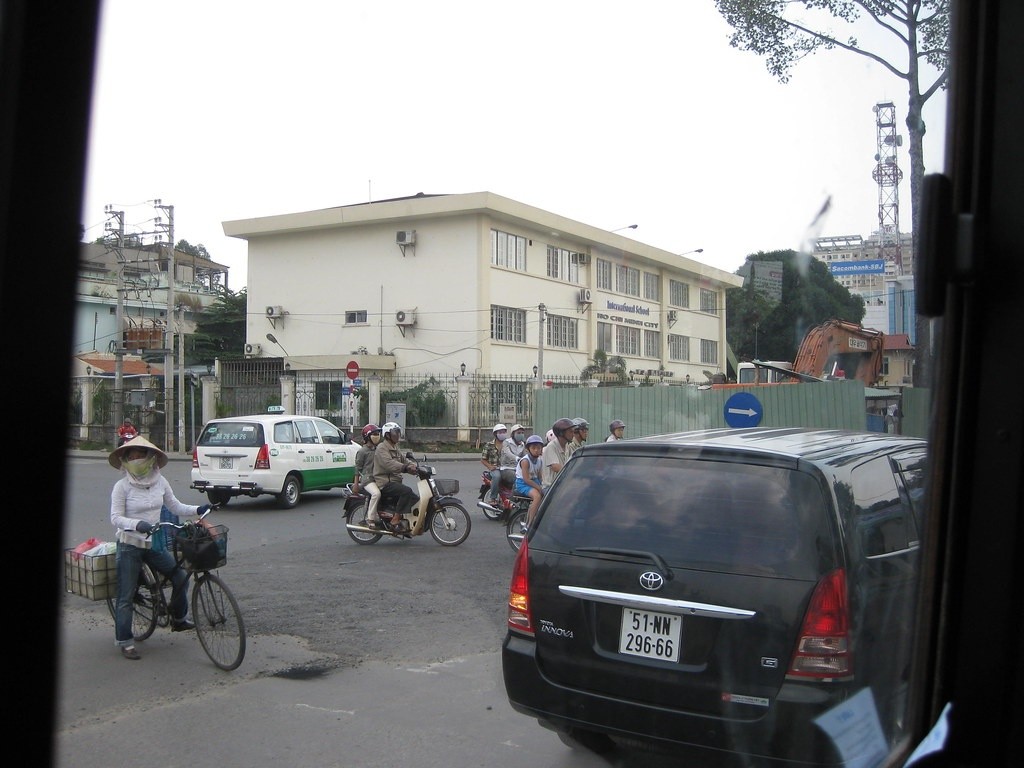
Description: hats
xmin=123 ymin=418 xmax=131 ymax=423
xmin=108 ymin=435 xmax=168 ymax=472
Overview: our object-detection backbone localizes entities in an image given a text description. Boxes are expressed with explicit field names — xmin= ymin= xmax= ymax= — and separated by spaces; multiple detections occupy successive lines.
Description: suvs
xmin=499 ymin=428 xmax=932 ymax=768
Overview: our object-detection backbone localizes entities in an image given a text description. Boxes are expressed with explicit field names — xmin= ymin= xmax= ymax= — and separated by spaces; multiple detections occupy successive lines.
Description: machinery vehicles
xmin=709 ymin=318 xmax=885 ymax=389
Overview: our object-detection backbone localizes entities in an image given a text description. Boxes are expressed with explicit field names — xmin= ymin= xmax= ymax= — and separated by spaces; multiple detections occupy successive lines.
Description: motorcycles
xmin=477 ymin=470 xmax=524 ymax=521
xmin=342 ymin=451 xmax=472 ymax=546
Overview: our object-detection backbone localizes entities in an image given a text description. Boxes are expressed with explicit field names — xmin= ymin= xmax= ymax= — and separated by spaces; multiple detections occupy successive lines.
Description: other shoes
xmin=171 ymin=620 xmax=195 ymax=632
xmin=521 ymin=523 xmax=529 ymax=531
xmin=490 ymin=500 xmax=498 ymax=506
xmin=367 ymin=519 xmax=377 ymax=529
xmin=121 ymin=646 xmax=141 ymax=660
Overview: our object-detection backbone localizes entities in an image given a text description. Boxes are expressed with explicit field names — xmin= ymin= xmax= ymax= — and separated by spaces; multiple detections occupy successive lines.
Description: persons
xmin=353 ymin=424 xmax=383 ymax=523
xmin=372 ymin=422 xmax=418 ymax=531
xmin=108 ymin=436 xmax=212 ymax=659
xmin=605 ymin=419 xmax=626 ymax=442
xmin=117 ymin=417 xmax=137 ymax=446
xmin=480 ymin=417 xmax=589 ymax=533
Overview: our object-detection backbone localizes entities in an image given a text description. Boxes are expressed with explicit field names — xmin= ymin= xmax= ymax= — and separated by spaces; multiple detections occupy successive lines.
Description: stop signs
xmin=345 ymin=361 xmax=361 ymax=382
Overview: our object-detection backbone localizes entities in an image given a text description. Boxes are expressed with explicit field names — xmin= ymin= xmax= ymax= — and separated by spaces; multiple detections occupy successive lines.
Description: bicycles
xmin=106 ymin=506 xmax=248 ymax=670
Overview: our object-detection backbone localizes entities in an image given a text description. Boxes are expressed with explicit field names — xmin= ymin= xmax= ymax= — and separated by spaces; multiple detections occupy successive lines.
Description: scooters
xmin=118 ymin=428 xmax=139 ymax=447
xmin=505 ymin=489 xmax=540 ymax=555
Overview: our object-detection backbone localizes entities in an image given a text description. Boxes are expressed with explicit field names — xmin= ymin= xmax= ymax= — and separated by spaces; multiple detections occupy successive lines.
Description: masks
xmin=125 ymin=457 xmax=150 ymax=475
xmin=368 ymin=434 xmax=380 ymax=443
xmin=496 ymin=433 xmax=508 ymax=441
xmin=513 ymin=434 xmax=524 ymax=442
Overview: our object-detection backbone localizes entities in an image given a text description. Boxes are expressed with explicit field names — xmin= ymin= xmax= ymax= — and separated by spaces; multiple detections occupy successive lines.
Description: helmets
xmin=382 ymin=422 xmax=400 ymax=437
xmin=610 ymin=420 xmax=626 ymax=431
xmin=492 ymin=423 xmax=507 ymax=434
xmin=362 ymin=423 xmax=382 ymax=441
xmin=572 ymin=418 xmax=590 ymax=431
xmin=511 ymin=424 xmax=524 ymax=435
xmin=525 ymin=435 xmax=543 ymax=448
xmin=553 ymin=417 xmax=577 ymax=436
xmin=545 ymin=429 xmax=557 ymax=442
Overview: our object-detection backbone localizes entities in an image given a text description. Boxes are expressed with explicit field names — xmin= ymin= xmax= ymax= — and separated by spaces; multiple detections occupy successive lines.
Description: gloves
xmin=196 ymin=504 xmax=214 ymax=515
xmin=136 ymin=520 xmax=153 ymax=534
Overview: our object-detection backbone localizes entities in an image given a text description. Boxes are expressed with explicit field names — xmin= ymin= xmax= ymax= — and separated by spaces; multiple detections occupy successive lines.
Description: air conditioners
xmin=579 ymin=288 xmax=592 ymax=302
xmin=668 ymin=310 xmax=679 ymax=320
xmin=395 ymin=309 xmax=415 ymax=326
xmin=244 ymin=343 xmax=260 ymax=355
xmin=579 ymin=253 xmax=591 ymax=264
xmin=395 ymin=229 xmax=416 ymax=246
xmin=267 ymin=304 xmax=283 ymax=320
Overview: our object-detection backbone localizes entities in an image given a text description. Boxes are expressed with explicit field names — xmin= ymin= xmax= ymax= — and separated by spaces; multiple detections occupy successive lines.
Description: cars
xmin=190 ymin=406 xmax=368 ymax=507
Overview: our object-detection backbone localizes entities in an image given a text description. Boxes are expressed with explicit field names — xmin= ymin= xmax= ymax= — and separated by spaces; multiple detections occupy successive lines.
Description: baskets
xmin=434 ymin=478 xmax=460 ymax=494
xmin=176 ymin=523 xmax=229 ymax=573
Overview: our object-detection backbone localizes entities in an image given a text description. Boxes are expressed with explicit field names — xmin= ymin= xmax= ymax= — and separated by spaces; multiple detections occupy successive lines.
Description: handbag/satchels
xmin=178 ymin=523 xmax=220 ymax=569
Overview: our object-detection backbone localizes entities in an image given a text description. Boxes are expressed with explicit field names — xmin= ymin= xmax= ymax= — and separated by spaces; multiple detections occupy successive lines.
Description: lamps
xmin=610 ymin=224 xmax=639 ymax=233
xmin=629 ymin=370 xmax=634 ymax=380
xmin=660 ymin=374 xmax=664 ymax=382
xmin=589 ymin=370 xmax=593 ymax=378
xmin=284 ymin=364 xmax=291 ymax=374
xmin=533 ymin=365 xmax=538 ymax=378
xmin=686 ymin=375 xmax=690 ymax=384
xmin=680 ymin=248 xmax=704 ymax=259
xmin=461 ymin=363 xmax=466 ymax=376
xmin=146 ymin=364 xmax=152 ymax=375
xmin=85 ymin=365 xmax=92 ymax=375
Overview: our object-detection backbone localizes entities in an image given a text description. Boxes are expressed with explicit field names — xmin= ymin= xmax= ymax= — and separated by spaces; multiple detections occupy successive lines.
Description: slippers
xmin=387 ymin=521 xmax=406 ymax=533
